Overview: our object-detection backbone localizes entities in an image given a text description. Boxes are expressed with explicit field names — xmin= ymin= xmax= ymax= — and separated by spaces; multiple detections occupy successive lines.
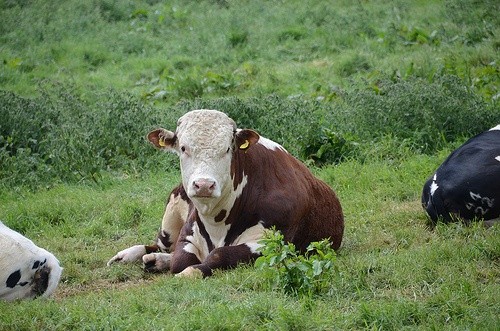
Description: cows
xmin=421 ymin=121 xmax=500 ymax=232
xmin=0 ymin=220 xmax=64 ymax=302
xmin=105 ymin=108 xmax=345 ymax=278
